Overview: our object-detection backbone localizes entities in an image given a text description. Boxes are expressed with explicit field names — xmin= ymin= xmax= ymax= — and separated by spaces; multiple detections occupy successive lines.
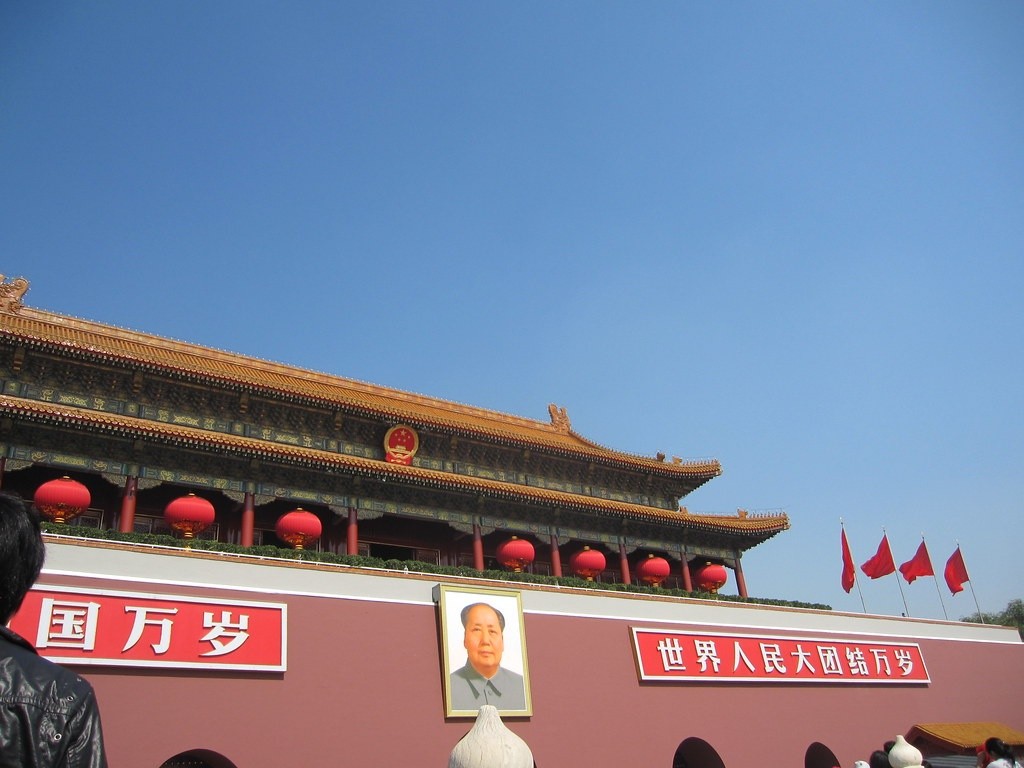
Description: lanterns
xmin=34 ymin=475 xmax=91 ymax=524
xmin=569 ymin=546 xmax=606 ymax=582
xmin=495 ymin=536 xmax=535 ymax=573
xmin=275 ymin=507 xmax=322 ymax=558
xmin=695 ymin=562 xmax=728 ymax=594
xmin=163 ymin=493 xmax=216 ymax=550
xmin=636 ymin=553 xmax=670 ymax=588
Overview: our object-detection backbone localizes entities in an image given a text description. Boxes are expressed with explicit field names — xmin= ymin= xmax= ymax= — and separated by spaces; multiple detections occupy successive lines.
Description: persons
xmin=0 ymin=491 xmax=111 ymax=768
xmin=450 ymin=603 xmax=525 ymax=712
xmin=870 ymin=735 xmax=1024 ymax=768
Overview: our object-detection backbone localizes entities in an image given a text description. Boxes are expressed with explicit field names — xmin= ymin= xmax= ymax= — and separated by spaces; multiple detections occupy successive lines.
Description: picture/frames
xmin=437 ymin=584 xmax=534 ymax=719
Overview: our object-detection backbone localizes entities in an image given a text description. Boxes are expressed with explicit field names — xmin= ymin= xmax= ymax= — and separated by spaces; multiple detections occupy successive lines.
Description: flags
xmin=899 ymin=541 xmax=934 ymax=585
xmin=943 ymin=548 xmax=969 ymax=597
xmin=860 ymin=535 xmax=895 ymax=579
xmin=841 ymin=530 xmax=855 ymax=593
